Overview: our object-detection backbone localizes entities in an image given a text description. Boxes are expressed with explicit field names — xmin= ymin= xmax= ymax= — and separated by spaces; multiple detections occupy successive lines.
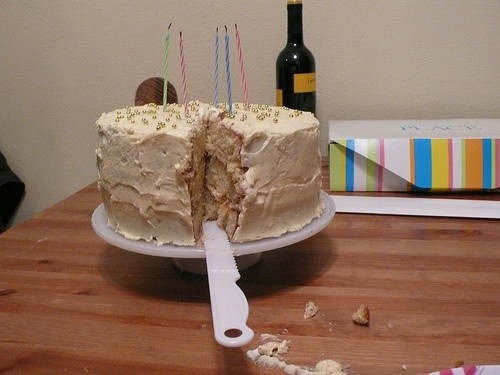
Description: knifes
xmin=202 ymin=215 xmax=254 ymax=348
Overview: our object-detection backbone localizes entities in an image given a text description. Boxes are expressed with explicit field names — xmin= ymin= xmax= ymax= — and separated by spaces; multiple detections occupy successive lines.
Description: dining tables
xmin=1 ymin=156 xmax=500 ymax=374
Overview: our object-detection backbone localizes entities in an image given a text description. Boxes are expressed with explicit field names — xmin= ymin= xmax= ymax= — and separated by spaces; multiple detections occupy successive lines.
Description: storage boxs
xmin=330 ymin=117 xmax=500 ymax=191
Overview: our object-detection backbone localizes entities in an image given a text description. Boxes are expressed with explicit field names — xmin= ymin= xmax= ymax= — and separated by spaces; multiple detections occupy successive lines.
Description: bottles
xmin=276 ymin=0 xmax=318 ymax=117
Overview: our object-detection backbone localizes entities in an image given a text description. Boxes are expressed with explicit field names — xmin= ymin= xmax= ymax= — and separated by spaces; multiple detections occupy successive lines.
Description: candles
xmin=162 ymin=22 xmax=175 ymax=109
xmin=178 ymin=31 xmax=191 ymax=111
xmin=222 ymin=23 xmax=233 ymax=110
xmin=215 ymin=27 xmax=224 ymax=107
xmin=234 ymin=24 xmax=251 ymax=107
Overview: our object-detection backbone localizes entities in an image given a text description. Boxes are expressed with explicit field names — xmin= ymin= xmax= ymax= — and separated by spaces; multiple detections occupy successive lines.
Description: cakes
xmin=95 ymin=100 xmax=326 ymax=246
